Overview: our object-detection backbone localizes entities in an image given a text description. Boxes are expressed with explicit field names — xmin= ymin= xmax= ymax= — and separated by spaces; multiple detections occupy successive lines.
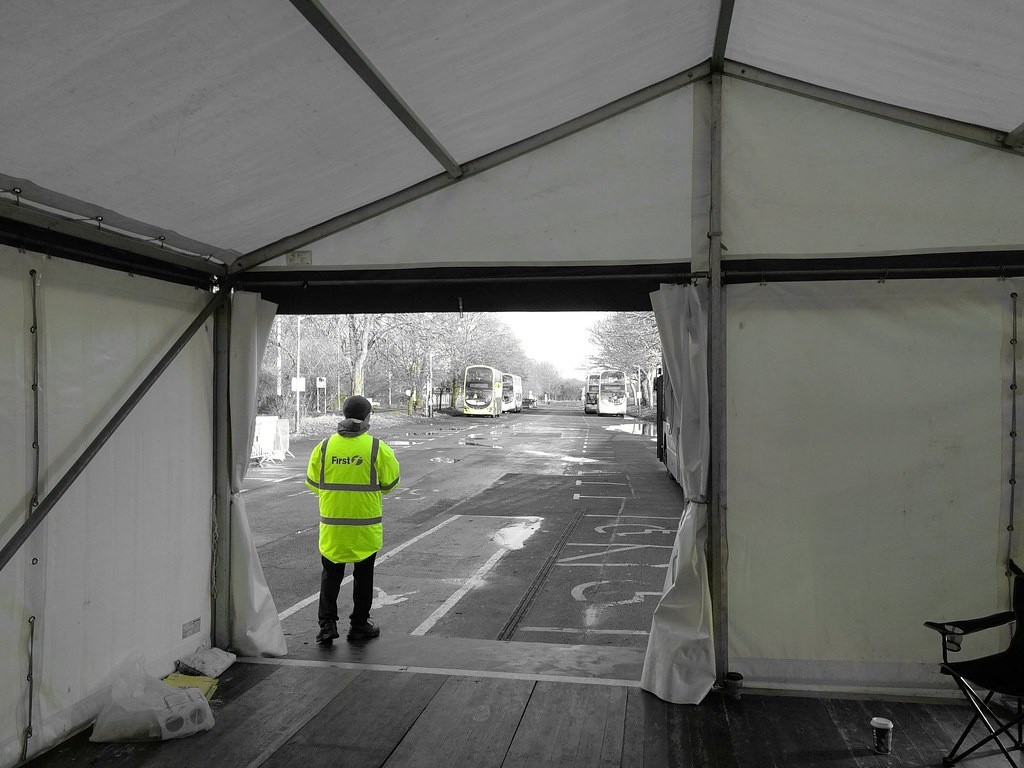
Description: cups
xmin=870 ymin=717 xmax=894 ymax=756
xmin=725 ymin=672 xmax=743 ymax=700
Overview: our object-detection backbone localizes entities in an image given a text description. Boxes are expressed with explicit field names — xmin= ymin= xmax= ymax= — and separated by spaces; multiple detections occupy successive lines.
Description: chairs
xmin=922 ymin=559 xmax=1024 ymax=768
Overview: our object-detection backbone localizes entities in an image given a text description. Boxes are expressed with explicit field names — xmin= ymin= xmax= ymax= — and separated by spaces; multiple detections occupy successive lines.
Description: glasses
xmin=369 ymin=411 xmax=374 ymax=415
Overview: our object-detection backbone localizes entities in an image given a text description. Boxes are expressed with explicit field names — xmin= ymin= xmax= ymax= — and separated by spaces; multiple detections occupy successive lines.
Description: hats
xmin=342 ymin=395 xmax=371 ymax=421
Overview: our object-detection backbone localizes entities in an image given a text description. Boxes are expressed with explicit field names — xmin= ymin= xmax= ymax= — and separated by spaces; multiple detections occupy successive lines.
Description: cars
xmin=522 ymin=398 xmax=537 ymax=409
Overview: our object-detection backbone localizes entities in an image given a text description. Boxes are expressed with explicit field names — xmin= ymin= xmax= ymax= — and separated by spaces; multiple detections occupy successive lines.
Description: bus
xmin=598 ymin=370 xmax=627 ymax=417
xmin=502 ymin=373 xmax=522 ymax=413
xmin=584 ymin=374 xmax=600 ymax=414
xmin=463 ymin=366 xmax=503 ymax=418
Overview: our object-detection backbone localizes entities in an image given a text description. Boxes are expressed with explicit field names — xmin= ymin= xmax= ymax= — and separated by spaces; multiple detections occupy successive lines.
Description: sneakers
xmin=316 ymin=620 xmax=339 ymax=642
xmin=347 ymin=623 xmax=380 ymax=640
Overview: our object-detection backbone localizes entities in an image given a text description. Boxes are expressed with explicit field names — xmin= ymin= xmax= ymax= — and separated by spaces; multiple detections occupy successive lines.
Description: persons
xmin=305 ymin=396 xmax=400 ymax=644
